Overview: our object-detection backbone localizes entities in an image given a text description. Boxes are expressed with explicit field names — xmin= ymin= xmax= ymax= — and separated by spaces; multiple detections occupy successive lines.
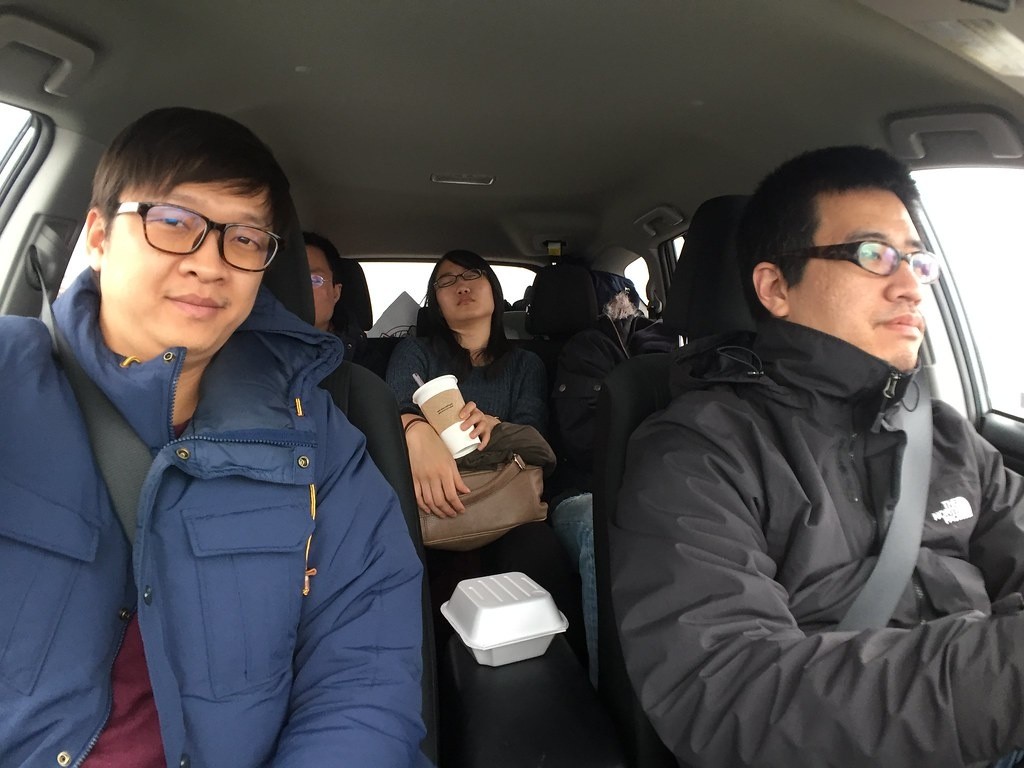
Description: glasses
xmin=310 ymin=275 xmax=334 ymax=287
xmin=115 ymin=201 xmax=281 ymax=274
xmin=432 ymin=267 xmax=483 ymax=288
xmin=773 ymin=240 xmax=942 ymax=285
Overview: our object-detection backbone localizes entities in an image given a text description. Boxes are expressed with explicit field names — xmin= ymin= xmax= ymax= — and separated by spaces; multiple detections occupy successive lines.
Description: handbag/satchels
xmin=416 ymin=453 xmax=548 ymax=551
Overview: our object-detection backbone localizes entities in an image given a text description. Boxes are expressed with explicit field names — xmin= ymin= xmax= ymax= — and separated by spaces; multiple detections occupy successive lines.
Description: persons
xmin=611 ymin=147 xmax=1024 ymax=768
xmin=303 ymin=231 xmax=373 ymax=372
xmin=0 ymin=107 xmax=423 ymax=768
xmin=387 ymin=250 xmax=588 ymax=658
xmin=547 ymin=294 xmax=670 ymax=686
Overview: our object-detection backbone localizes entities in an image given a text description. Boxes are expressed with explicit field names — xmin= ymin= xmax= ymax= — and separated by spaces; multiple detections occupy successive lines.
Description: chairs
xmin=331 ymin=256 xmax=407 ymax=367
xmin=505 ymin=301 xmax=537 ymax=350
xmin=592 ymin=193 xmax=752 ymax=768
xmin=261 ymin=153 xmax=438 ymax=768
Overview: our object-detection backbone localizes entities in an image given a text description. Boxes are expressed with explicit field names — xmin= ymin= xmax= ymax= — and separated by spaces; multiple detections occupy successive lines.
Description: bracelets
xmin=404 ymin=418 xmax=427 ymax=433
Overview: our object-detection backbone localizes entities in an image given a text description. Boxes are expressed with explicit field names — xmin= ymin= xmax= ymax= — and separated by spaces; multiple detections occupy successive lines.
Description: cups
xmin=411 ymin=374 xmax=480 ymax=459
